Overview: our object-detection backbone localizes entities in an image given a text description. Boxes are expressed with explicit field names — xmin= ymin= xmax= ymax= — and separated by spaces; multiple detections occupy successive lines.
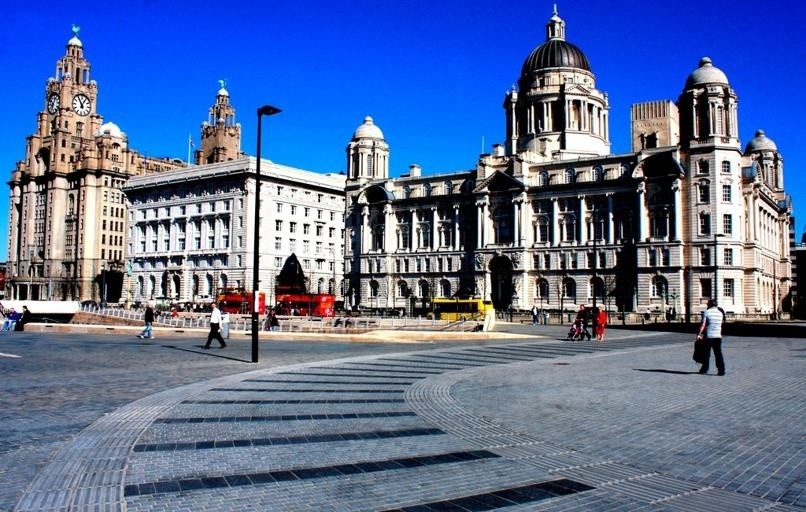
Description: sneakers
xmin=139 ymin=334 xmax=155 ymax=339
xmin=699 ymin=369 xmax=724 ymax=376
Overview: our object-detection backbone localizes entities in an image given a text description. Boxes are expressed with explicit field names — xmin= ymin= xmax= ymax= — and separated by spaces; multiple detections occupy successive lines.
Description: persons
xmin=13 ymin=306 xmax=32 ymax=331
xmin=154 ymin=301 xmax=208 ymax=319
xmin=646 ymin=306 xmax=674 ymax=322
xmin=570 ymin=323 xmax=577 ymax=337
xmin=290 ymin=305 xmax=310 ymax=315
xmin=138 ymin=303 xmax=155 ymax=340
xmin=696 ymin=300 xmax=726 ymax=377
xmin=399 ymin=309 xmax=403 ymax=319
xmin=532 ymin=306 xmax=540 ymax=326
xmin=261 ymin=305 xmax=279 ymax=331
xmin=594 ymin=305 xmax=607 ymax=341
xmin=1 ymin=308 xmax=21 ymax=331
xmin=575 ymin=304 xmax=591 ymax=342
xmin=542 ymin=311 xmax=548 ymax=325
xmin=202 ymin=303 xmax=227 ymax=350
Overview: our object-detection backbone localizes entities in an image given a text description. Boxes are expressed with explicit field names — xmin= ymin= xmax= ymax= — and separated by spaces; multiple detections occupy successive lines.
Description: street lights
xmin=251 ymin=105 xmax=283 ymax=363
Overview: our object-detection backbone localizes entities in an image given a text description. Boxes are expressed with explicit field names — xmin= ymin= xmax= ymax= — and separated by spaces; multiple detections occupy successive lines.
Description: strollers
xmin=567 ymin=317 xmax=587 ymax=340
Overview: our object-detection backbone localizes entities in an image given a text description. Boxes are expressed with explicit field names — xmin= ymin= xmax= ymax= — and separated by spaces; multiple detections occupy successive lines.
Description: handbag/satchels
xmin=692 ymin=339 xmax=705 ymax=364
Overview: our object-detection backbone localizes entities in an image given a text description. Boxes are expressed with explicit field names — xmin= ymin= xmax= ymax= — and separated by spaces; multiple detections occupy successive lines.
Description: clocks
xmin=72 ymin=93 xmax=92 ymax=116
xmin=47 ymin=92 xmax=61 ymax=115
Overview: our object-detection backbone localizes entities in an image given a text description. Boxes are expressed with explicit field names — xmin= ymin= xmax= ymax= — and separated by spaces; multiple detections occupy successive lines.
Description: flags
xmin=190 ymin=137 xmax=195 ymax=148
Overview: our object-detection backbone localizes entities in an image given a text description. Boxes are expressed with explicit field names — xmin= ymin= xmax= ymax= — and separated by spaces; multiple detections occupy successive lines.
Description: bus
xmin=214 ymin=292 xmax=265 ymax=315
xmin=426 ymin=297 xmax=494 ymax=321
xmin=274 ymin=294 xmax=335 ymax=316
